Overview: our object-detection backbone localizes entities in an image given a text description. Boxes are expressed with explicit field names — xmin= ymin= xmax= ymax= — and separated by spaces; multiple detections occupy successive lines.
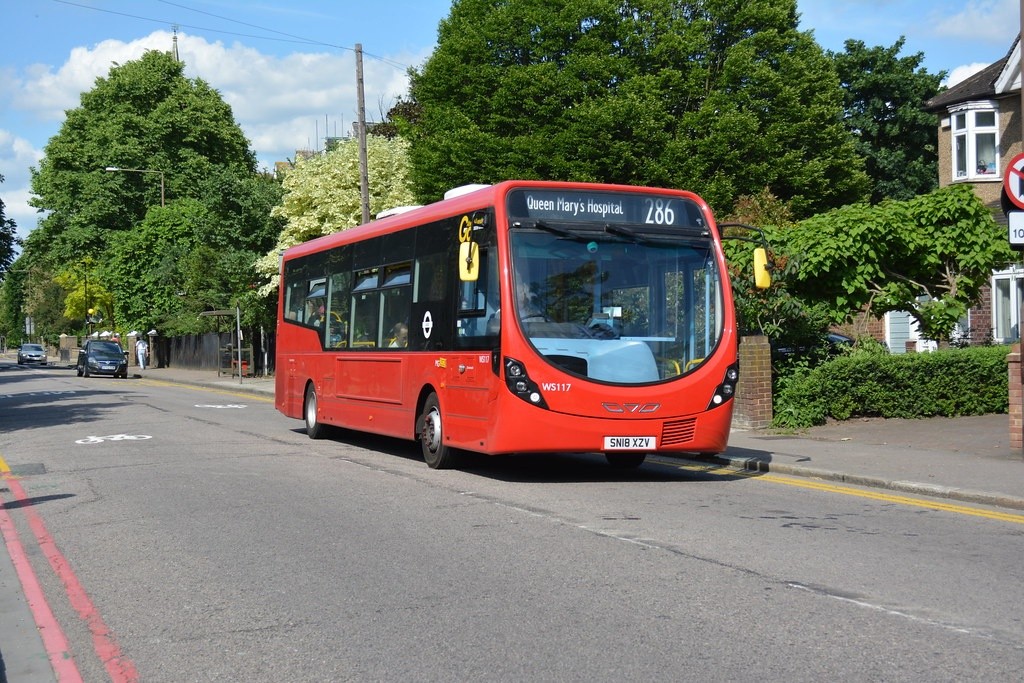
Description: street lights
xmin=104 ymin=166 xmax=166 ymax=207
xmin=80 ymin=261 xmax=88 ymax=339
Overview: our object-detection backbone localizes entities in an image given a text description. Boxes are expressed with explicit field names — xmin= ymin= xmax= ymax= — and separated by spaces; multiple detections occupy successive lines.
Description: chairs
xmin=459 ymin=258 xmax=523 ymax=336
xmin=321 ymin=312 xmax=408 ymax=347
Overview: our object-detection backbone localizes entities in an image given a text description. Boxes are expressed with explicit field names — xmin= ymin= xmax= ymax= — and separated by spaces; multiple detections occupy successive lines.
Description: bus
xmin=274 ymin=179 xmax=771 ymax=470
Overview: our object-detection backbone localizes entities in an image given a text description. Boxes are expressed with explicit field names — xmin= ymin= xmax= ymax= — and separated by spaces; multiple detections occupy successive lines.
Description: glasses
xmin=580 ymin=279 xmax=592 ymax=284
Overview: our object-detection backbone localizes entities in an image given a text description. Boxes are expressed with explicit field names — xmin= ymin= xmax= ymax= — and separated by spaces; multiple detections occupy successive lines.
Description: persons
xmin=111 ymin=335 xmax=120 ymax=345
xmin=389 ymin=322 xmax=409 ymax=348
xmin=308 ymin=297 xmax=344 ymax=347
xmin=493 ymin=287 xmax=546 ymax=334
xmin=134 ymin=336 xmax=149 ymax=369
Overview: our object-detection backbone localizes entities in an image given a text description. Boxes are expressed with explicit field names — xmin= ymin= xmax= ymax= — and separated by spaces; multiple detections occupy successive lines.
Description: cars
xmin=777 ymin=332 xmax=855 ymax=356
xmin=17 ymin=343 xmax=48 ymax=365
xmin=77 ymin=339 xmax=129 ymax=379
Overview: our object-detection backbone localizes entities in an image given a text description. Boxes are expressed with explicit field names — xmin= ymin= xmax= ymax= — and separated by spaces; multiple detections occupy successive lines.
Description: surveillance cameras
xmin=587 ymin=242 xmax=598 ymax=253
